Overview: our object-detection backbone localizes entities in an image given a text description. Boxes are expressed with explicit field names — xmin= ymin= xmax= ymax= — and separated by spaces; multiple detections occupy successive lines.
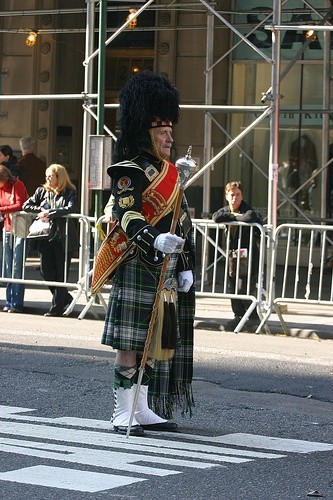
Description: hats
xmin=111 ymin=72 xmax=179 ymax=162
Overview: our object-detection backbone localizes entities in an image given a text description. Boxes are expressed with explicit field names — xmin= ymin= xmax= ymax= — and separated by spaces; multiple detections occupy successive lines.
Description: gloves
xmin=178 ymin=270 xmax=192 ymax=294
xmin=154 ymin=232 xmax=183 ymax=253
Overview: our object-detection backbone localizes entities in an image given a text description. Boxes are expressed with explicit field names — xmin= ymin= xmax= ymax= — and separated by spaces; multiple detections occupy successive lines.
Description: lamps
xmin=25 ymin=30 xmax=39 ymax=47
xmin=125 ymin=8 xmax=138 ymax=30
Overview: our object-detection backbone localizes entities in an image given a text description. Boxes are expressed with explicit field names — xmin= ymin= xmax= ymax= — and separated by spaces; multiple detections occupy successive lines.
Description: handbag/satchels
xmin=26 ymin=215 xmax=53 ymax=240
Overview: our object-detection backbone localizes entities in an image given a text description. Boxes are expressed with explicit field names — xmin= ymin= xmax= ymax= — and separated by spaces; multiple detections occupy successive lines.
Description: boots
xmin=132 ymin=384 xmax=177 ymax=431
xmin=111 ymin=386 xmax=142 ymax=436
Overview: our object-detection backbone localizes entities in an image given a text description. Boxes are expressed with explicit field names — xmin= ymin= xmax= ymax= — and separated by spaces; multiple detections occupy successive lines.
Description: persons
xmin=22 ymin=164 xmax=81 ymax=317
xmin=0 ymin=135 xmax=46 ymax=313
xmin=289 ymin=134 xmax=318 ymax=217
xmin=213 ymin=181 xmax=263 ymax=333
xmin=102 ymin=71 xmax=196 ymax=437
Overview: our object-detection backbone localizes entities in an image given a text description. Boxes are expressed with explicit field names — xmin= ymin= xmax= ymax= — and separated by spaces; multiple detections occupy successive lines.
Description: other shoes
xmin=42 ymin=295 xmax=73 ymax=316
xmin=226 ymin=316 xmax=260 ymax=327
xmin=2 ymin=305 xmax=18 ymax=313
